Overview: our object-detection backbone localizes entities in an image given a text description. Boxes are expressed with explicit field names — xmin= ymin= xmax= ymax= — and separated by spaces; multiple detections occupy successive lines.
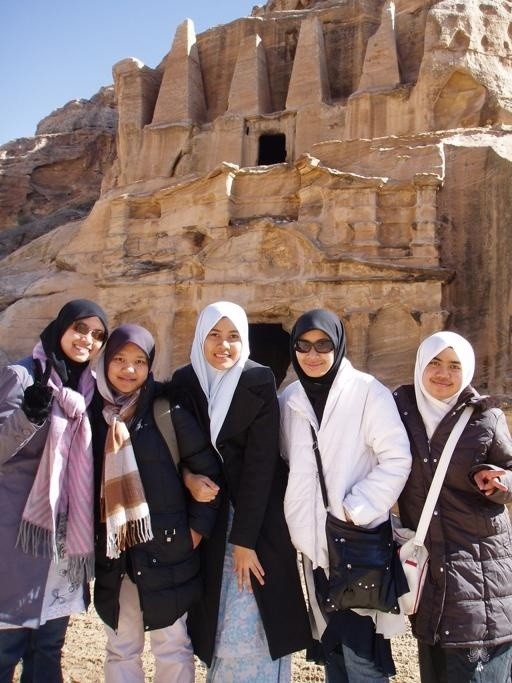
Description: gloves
xmin=22 ymin=358 xmax=54 ymax=422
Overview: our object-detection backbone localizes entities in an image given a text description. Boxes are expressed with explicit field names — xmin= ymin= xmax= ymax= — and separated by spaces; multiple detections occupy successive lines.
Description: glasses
xmin=293 ymin=338 xmax=334 ymax=352
xmin=72 ymin=320 xmax=106 ymax=340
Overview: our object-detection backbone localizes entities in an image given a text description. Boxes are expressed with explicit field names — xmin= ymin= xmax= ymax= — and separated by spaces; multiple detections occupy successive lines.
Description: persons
xmin=173 ymin=300 xmax=311 ymax=683
xmin=90 ymin=323 xmax=223 ymax=682
xmin=274 ymin=311 xmax=415 ymax=682
xmin=391 ymin=330 xmax=509 ymax=682
xmin=3 ymin=297 xmax=109 ymax=682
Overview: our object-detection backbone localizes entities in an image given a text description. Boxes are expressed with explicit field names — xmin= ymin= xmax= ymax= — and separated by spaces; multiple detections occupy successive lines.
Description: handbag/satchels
xmin=322 ymin=512 xmax=400 ymax=615
xmin=392 ymin=527 xmax=430 ymax=616
